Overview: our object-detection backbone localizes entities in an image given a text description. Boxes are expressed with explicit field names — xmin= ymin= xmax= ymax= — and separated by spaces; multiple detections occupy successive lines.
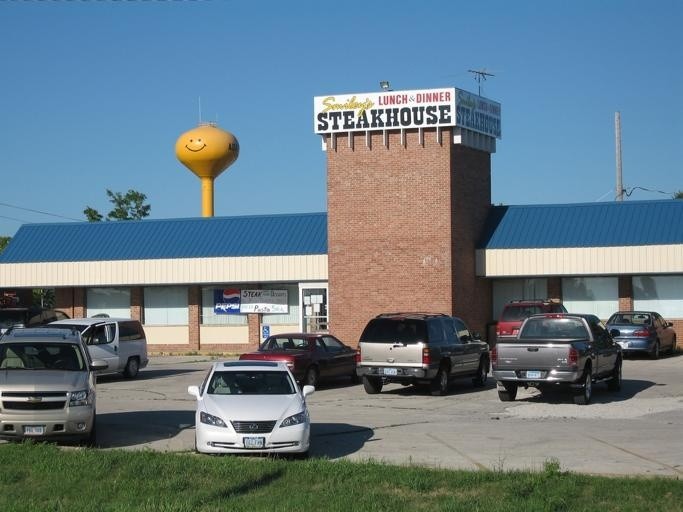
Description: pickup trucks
xmin=492 ymin=312 xmax=623 ymax=405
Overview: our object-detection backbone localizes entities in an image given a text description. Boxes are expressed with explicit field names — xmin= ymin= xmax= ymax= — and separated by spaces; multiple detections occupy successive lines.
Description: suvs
xmin=356 ymin=311 xmax=490 ymax=395
xmin=495 ymin=298 xmax=569 ymax=337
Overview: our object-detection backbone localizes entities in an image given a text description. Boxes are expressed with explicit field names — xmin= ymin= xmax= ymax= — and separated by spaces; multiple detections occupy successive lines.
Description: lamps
xmin=380 ymin=81 xmax=390 ymax=92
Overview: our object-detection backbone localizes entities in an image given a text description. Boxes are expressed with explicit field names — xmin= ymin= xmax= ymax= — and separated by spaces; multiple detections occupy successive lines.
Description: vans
xmin=0 ymin=307 xmax=148 ymax=446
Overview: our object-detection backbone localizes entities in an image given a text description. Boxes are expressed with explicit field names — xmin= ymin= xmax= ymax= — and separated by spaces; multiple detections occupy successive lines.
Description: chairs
xmin=211 ymin=375 xmax=239 ymax=394
xmin=1 ymin=347 xmax=29 ymax=369
xmin=621 ymin=319 xmax=629 ymax=324
xmin=282 ymin=342 xmax=294 ymax=348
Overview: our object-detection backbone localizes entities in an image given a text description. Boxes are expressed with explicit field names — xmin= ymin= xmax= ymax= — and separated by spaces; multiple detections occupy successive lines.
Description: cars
xmin=188 ymin=360 xmax=315 ymax=455
xmin=239 ymin=333 xmax=357 ymax=390
xmin=605 ymin=310 xmax=677 ymax=360
